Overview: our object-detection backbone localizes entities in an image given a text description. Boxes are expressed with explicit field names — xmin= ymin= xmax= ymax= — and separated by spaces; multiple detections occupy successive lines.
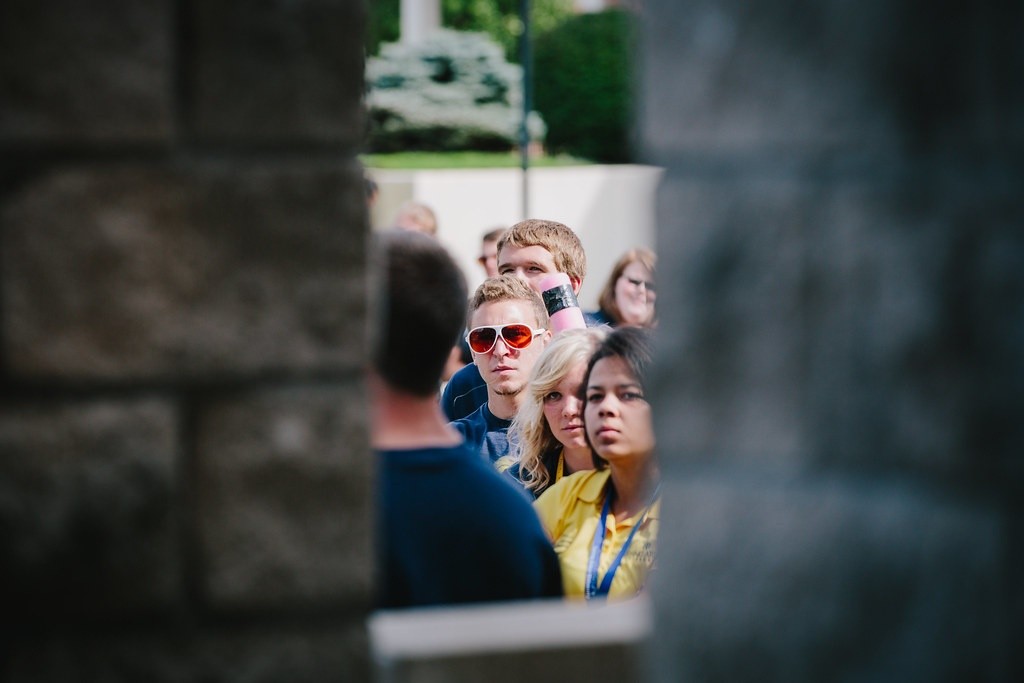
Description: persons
xmin=365 ymin=229 xmax=565 ymax=615
xmin=438 ymin=219 xmax=663 ymax=607
xmin=366 ymin=179 xmax=436 ymax=236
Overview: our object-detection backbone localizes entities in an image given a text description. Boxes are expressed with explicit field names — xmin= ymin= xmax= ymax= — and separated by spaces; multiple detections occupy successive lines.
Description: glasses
xmin=465 ymin=324 xmax=546 ymax=354
xmin=620 ymin=274 xmax=656 ymax=290
